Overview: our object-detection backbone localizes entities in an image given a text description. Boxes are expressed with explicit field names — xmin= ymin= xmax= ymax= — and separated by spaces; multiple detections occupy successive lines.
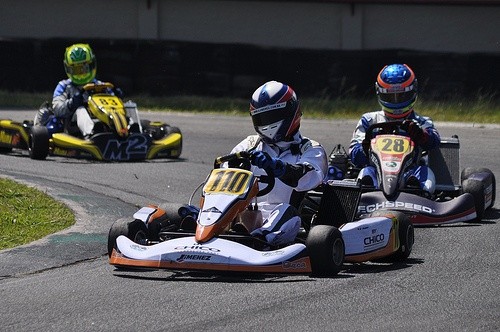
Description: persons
xmin=349 ymin=63 xmax=441 ymax=196
xmin=45 ymin=42 xmax=145 ymax=142
xmin=221 ymin=80 xmax=330 ymax=250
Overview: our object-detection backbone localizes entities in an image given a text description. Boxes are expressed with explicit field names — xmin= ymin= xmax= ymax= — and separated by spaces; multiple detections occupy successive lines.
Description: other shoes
xmin=180 ymin=216 xmax=197 ymax=230
xmin=405 ymin=176 xmax=419 ymax=189
xmin=361 ymin=175 xmax=373 ymax=185
xmin=232 ymin=222 xmax=250 ymax=235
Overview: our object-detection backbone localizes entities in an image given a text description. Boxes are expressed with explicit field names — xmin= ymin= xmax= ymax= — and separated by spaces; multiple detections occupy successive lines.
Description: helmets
xmin=249 ymin=81 xmax=302 ymax=144
xmin=375 ymin=64 xmax=418 ymax=119
xmin=64 ymin=44 xmax=97 ymax=84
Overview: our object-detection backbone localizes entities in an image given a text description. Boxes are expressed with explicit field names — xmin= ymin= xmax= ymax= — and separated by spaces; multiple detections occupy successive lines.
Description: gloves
xmin=69 ymin=89 xmax=90 ymax=108
xmin=400 ymin=120 xmax=430 ymax=142
xmin=248 ymin=151 xmax=286 ymax=179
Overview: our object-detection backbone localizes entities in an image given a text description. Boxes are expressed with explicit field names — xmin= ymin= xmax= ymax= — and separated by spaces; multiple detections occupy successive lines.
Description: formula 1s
xmin=0 ymin=83 xmax=183 ymax=161
xmin=326 ymin=122 xmax=497 ymax=226
xmin=108 ymin=151 xmax=414 ymax=275
